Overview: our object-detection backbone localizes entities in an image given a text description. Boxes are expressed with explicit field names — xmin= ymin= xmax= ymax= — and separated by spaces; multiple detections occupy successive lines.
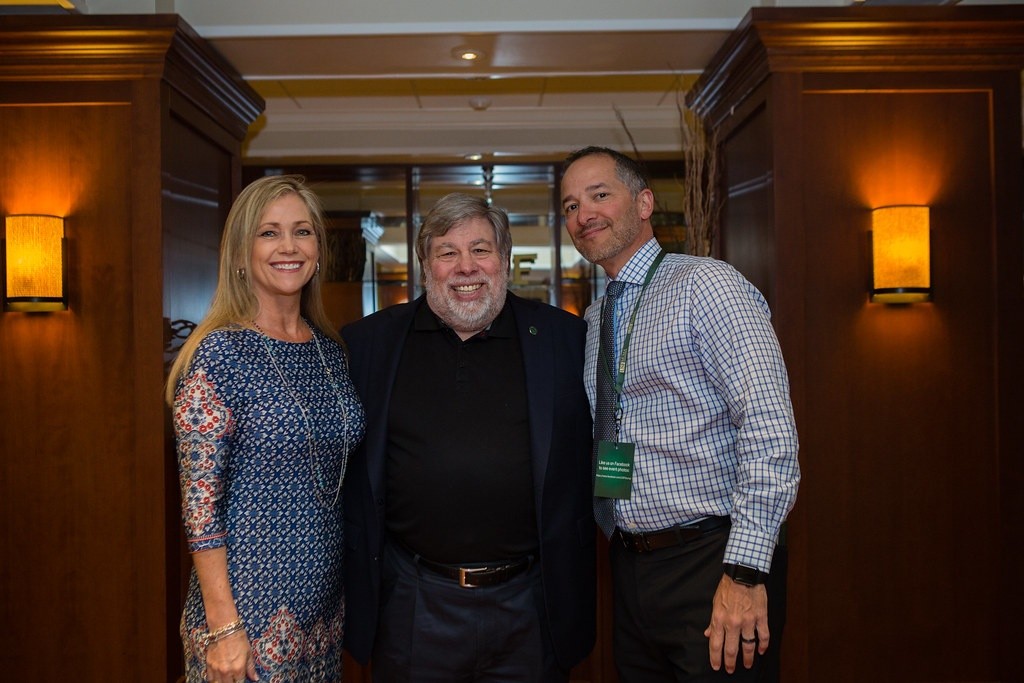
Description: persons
xmin=166 ymin=176 xmax=366 ymax=683
xmin=337 ymin=192 xmax=597 ymax=683
xmin=558 ymin=148 xmax=801 ymax=683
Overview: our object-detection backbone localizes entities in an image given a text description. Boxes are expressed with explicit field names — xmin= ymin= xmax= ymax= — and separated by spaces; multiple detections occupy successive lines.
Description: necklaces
xmin=249 ymin=316 xmax=348 ymax=509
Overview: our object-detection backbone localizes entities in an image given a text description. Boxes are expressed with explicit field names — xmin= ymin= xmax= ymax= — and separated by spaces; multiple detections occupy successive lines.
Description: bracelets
xmin=722 ymin=562 xmax=770 ymax=587
xmin=202 ymin=618 xmax=245 ymax=645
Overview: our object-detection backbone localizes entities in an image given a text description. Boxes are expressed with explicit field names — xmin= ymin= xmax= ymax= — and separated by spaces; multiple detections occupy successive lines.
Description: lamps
xmin=870 ymin=205 xmax=932 ymax=304
xmin=3 ymin=215 xmax=68 ymax=313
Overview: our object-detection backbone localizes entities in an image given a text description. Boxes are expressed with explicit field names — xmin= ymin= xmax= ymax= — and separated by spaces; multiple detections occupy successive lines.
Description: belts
xmin=616 ymin=514 xmax=730 ymax=554
xmin=394 ymin=546 xmax=540 ymax=588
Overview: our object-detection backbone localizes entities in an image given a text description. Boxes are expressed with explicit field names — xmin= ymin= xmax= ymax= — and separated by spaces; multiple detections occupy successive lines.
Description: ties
xmin=593 ymin=280 xmax=626 ymax=538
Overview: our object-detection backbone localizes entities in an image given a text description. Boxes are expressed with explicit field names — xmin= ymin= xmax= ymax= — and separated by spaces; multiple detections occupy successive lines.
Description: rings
xmin=742 ymin=637 xmax=755 ymax=642
